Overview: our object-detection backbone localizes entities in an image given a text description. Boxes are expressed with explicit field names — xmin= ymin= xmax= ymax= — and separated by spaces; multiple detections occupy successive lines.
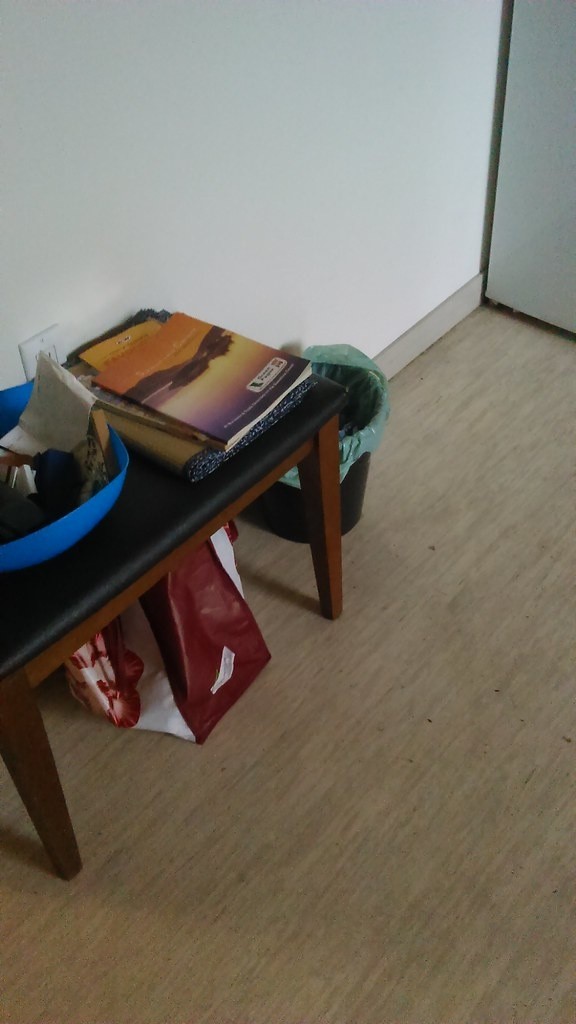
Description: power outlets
xmin=18 ymin=324 xmax=67 ymax=382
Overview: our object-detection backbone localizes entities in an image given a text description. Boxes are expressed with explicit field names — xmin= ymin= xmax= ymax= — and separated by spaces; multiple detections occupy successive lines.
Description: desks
xmin=0 ymin=373 xmax=351 ymax=881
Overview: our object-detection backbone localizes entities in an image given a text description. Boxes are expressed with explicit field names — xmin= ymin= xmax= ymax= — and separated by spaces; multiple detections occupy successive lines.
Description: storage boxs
xmin=0 ymin=390 xmax=128 ymax=572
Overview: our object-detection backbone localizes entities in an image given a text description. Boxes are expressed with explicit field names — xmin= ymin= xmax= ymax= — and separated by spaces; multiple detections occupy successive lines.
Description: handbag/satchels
xmin=65 ymin=517 xmax=271 ymax=748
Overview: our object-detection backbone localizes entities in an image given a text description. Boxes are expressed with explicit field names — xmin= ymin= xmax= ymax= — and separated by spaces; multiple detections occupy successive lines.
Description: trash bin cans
xmin=259 ymin=341 xmax=390 ymax=545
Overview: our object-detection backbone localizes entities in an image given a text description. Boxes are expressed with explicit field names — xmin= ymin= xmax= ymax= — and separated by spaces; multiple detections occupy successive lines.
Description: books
xmin=57 ymin=302 xmax=312 ymax=455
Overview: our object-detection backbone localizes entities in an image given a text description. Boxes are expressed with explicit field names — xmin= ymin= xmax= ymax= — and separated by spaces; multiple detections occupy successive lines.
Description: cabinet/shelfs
xmin=485 ymin=0 xmax=575 ymax=335
